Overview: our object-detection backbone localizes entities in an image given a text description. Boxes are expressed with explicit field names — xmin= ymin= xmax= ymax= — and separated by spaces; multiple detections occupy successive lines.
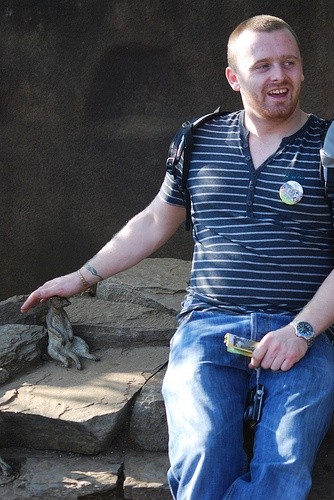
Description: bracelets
xmin=76 ymin=270 xmax=92 ymax=289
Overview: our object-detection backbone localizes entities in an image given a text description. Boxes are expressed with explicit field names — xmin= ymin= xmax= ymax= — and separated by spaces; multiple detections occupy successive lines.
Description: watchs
xmin=289 ymin=319 xmax=316 ymax=348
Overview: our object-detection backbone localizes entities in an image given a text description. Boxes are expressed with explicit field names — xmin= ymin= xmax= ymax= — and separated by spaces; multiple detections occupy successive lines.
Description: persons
xmin=18 ymin=14 xmax=334 ymax=500
